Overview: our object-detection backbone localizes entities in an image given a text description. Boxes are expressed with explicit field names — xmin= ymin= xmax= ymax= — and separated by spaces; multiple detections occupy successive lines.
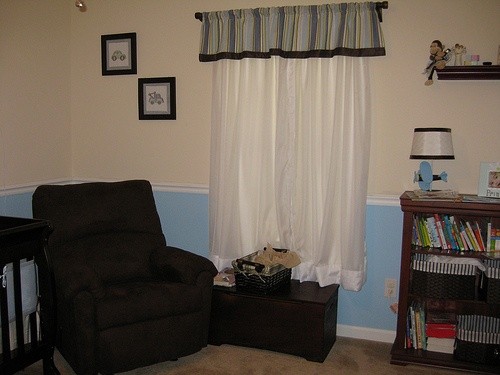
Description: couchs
xmin=32 ymin=179 xmax=218 ymax=375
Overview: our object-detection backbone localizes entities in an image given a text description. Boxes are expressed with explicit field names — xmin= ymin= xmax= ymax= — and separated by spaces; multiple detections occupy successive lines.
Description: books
xmin=213 ymin=272 xmax=236 ymax=288
xmin=406 ymin=299 xmax=459 ymax=354
xmin=412 ymin=213 xmax=500 ymax=252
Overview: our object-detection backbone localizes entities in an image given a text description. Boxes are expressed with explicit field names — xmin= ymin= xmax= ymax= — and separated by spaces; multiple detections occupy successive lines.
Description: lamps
xmin=409 ymin=127 xmax=455 ymax=192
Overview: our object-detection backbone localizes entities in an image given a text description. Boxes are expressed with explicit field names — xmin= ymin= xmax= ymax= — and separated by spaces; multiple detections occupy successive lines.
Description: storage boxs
xmin=411 ymin=254 xmax=478 ymax=300
xmin=482 ymin=259 xmax=500 ymax=303
xmin=456 ymin=315 xmax=499 ymax=365
xmin=233 ymin=247 xmax=292 ymax=296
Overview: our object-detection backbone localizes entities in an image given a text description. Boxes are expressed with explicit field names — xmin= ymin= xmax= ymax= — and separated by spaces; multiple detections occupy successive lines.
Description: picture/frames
xmin=101 ymin=32 xmax=137 ymax=76
xmin=138 ymin=77 xmax=176 ymax=120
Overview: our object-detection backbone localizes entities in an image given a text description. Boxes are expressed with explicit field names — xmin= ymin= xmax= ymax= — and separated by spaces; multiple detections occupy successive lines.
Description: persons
xmin=488 ymin=172 xmax=500 ymax=188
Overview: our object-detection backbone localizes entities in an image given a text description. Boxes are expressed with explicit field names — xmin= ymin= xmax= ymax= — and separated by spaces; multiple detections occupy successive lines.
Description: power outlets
xmin=383 ymin=279 xmax=396 ymax=297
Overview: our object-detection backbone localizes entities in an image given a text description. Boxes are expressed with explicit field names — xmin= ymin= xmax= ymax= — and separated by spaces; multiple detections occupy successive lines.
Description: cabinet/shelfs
xmin=390 ymin=191 xmax=499 ymax=375
xmin=208 ymin=279 xmax=340 ymax=363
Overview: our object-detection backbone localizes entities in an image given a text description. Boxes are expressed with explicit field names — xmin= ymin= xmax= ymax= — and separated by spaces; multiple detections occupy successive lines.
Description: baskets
xmin=234 ymin=247 xmax=292 ymax=296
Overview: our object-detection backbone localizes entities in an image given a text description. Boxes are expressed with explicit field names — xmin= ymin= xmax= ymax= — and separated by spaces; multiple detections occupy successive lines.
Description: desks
xmin=0 ymin=215 xmax=64 ymax=374
